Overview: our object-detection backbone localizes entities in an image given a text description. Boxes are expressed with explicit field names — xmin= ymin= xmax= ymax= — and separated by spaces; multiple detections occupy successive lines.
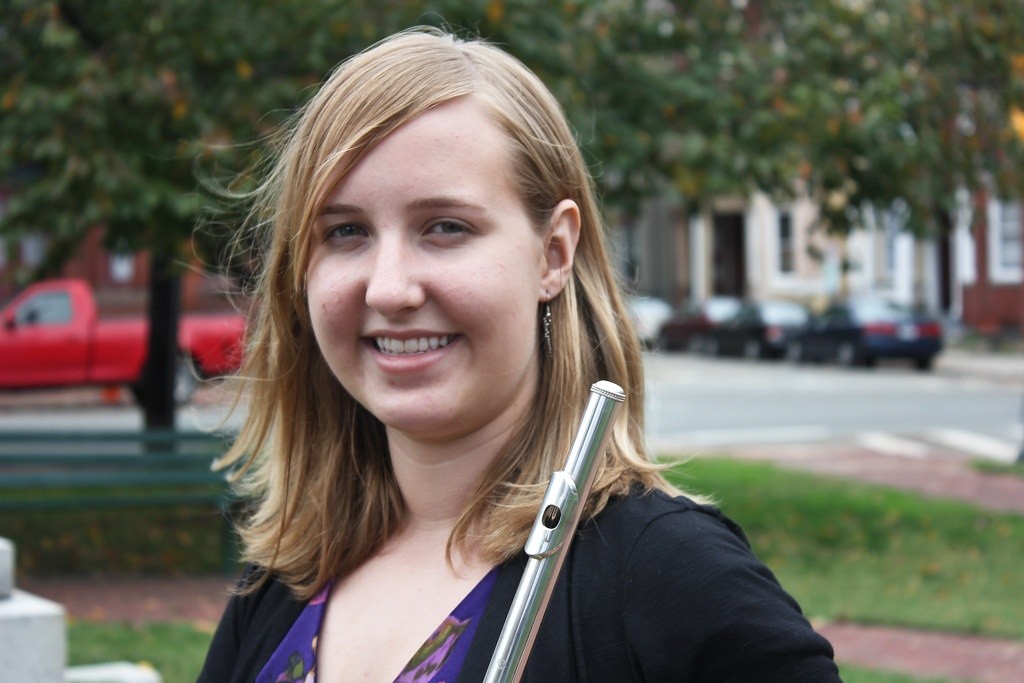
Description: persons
xmin=195 ymin=26 xmax=841 ymax=682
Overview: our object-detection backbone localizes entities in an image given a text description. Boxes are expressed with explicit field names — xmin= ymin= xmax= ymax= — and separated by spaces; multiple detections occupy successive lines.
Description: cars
xmin=788 ymin=294 xmax=944 ymax=371
xmin=621 ymin=295 xmax=811 ymax=362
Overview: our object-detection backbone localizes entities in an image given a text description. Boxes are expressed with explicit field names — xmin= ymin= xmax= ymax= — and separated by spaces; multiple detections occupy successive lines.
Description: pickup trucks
xmin=0 ymin=279 xmax=249 ymax=404
